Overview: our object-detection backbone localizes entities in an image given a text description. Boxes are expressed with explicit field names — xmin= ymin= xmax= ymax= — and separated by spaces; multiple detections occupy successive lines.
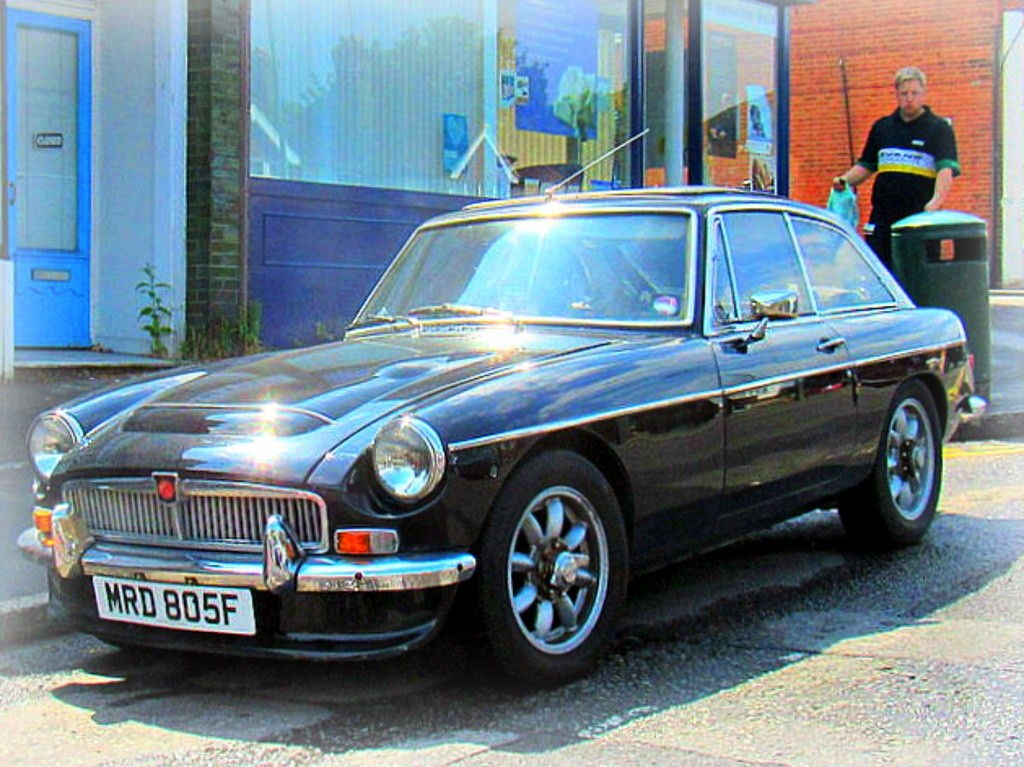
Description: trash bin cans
xmin=890 ymin=212 xmax=993 ymax=410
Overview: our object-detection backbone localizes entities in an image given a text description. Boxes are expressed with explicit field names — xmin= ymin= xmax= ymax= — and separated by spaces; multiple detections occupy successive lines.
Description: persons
xmin=834 ymin=67 xmax=959 ymax=261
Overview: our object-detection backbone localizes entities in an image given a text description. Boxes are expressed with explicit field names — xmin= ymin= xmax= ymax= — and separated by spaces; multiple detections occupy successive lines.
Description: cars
xmin=22 ymin=184 xmax=979 ymax=692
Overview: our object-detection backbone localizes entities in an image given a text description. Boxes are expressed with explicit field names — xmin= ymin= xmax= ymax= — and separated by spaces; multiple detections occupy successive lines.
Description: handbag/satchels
xmin=825 ymin=182 xmax=860 ymax=228
